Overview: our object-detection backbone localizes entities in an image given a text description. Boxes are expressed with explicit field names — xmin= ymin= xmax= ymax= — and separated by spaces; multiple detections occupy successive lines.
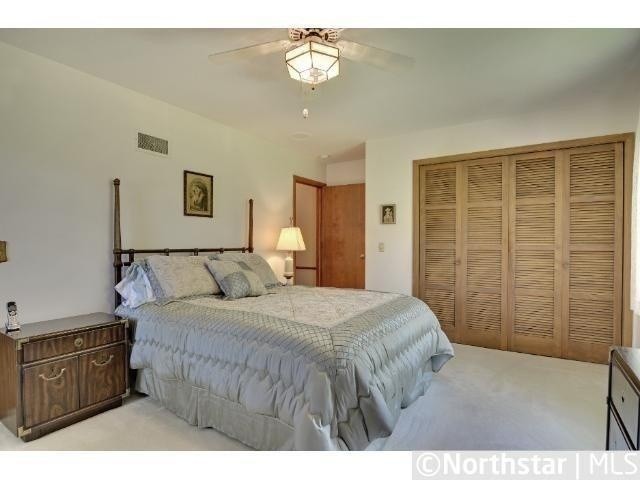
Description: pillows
xmin=204 ymin=253 xmax=283 ymax=289
xmin=114 ymin=262 xmax=156 ymax=308
xmin=132 ymin=255 xmax=222 ymax=307
xmin=204 ymin=258 xmax=269 ymax=301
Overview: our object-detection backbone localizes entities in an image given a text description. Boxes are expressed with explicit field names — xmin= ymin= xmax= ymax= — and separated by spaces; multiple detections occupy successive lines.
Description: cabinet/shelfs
xmin=606 ymin=345 xmax=640 ymax=451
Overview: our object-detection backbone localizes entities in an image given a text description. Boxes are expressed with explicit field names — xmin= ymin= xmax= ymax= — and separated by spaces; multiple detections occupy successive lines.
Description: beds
xmin=113 ymin=178 xmax=455 ymax=450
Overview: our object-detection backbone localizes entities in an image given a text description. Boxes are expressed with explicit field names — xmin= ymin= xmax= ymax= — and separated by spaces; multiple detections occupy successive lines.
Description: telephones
xmin=5 ymin=302 xmax=21 ymax=330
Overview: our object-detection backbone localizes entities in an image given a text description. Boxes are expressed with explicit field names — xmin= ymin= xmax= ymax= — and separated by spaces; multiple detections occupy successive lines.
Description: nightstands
xmin=0 ymin=312 xmax=130 ymax=442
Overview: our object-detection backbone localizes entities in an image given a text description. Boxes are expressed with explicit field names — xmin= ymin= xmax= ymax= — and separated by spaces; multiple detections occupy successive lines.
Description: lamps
xmin=285 ymin=28 xmax=345 ymax=91
xmin=276 ymin=217 xmax=306 ymax=286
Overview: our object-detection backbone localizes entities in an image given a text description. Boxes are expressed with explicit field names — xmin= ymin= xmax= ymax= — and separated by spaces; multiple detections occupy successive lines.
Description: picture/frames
xmin=380 ymin=204 xmax=396 ymax=224
xmin=184 ymin=170 xmax=213 ymax=218
